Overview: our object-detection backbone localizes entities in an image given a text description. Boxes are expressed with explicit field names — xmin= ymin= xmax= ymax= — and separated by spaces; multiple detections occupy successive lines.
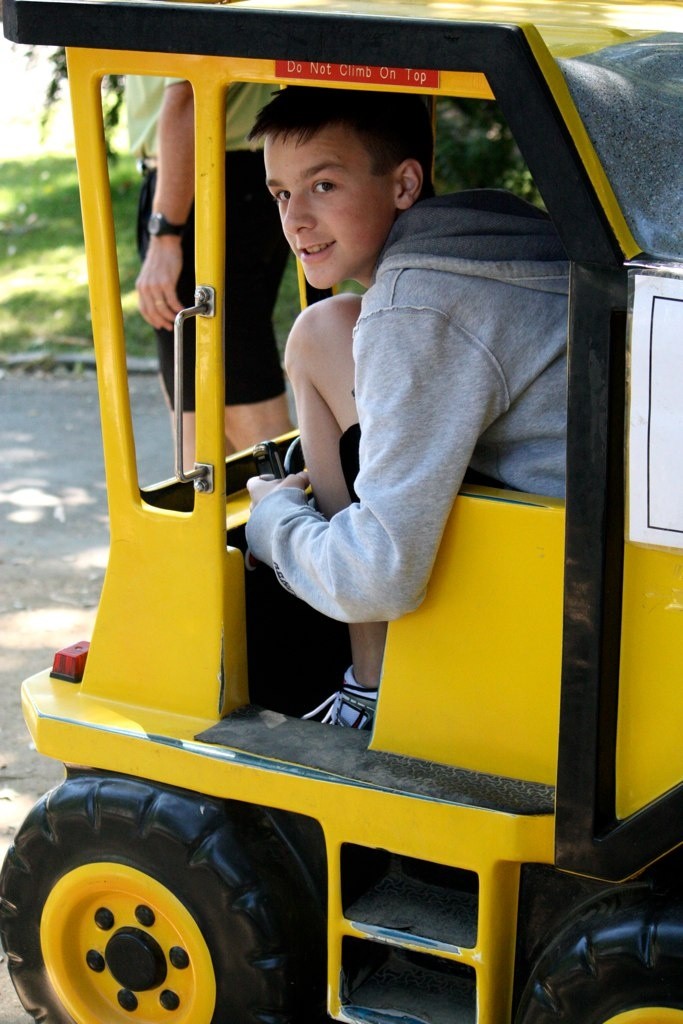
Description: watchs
xmin=148 ymin=213 xmax=185 ymax=236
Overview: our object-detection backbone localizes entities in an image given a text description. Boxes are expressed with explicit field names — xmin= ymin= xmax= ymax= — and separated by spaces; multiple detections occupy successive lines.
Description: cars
xmin=2 ymin=2 xmax=683 ymax=1024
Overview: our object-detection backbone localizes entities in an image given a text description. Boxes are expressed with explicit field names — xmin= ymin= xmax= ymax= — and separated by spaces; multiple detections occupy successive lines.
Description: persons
xmin=243 ymin=84 xmax=572 ymax=729
xmin=119 ymin=73 xmax=294 ymax=478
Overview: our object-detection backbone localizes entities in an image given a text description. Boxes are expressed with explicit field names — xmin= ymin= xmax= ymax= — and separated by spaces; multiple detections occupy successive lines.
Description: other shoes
xmin=300 ymin=663 xmax=378 ymax=733
